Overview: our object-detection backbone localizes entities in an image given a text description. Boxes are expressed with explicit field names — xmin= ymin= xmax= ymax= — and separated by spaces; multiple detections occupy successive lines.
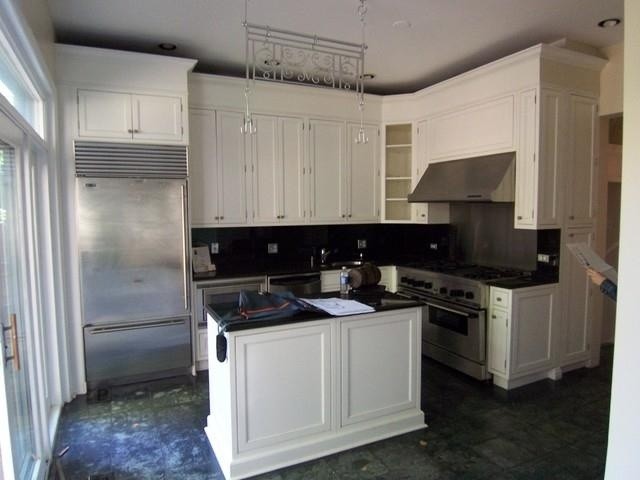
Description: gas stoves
xmin=397 ymin=256 xmax=525 ymax=309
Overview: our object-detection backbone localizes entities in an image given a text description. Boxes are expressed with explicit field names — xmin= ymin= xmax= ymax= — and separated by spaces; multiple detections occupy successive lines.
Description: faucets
xmin=319 ymin=247 xmax=331 ymax=267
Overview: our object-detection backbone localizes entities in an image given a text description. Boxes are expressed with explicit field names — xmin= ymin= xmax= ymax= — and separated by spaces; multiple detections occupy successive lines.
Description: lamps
xmin=232 ymin=2 xmax=373 ymax=147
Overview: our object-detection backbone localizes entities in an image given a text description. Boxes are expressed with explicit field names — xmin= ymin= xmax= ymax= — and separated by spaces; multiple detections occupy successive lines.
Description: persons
xmin=585 ymin=268 xmax=617 ymax=301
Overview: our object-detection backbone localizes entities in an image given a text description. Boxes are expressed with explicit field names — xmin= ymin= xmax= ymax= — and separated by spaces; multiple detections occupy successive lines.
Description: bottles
xmin=340 ymin=266 xmax=349 ymax=294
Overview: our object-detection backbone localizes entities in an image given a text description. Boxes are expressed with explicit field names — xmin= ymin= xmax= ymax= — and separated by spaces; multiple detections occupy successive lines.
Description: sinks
xmin=332 ymin=258 xmax=363 ymax=268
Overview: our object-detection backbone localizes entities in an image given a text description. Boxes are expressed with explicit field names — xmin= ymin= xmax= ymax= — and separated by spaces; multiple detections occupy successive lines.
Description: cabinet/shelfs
xmin=319 ymin=265 xmax=397 ymax=293
xmin=250 ymin=110 xmax=307 ymax=224
xmin=309 ymin=117 xmax=379 ymax=222
xmin=75 ymin=87 xmax=182 ymax=143
xmin=516 ymin=89 xmax=599 ymax=231
xmin=188 ymin=106 xmax=248 ymax=226
xmin=383 ymin=119 xmax=450 ymax=223
xmin=487 ymin=283 xmax=558 ymax=382
xmin=428 ymin=94 xmax=515 ymax=161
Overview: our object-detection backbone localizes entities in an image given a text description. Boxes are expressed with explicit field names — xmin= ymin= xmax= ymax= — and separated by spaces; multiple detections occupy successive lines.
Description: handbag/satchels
xmin=218 ymin=289 xmax=306 ymax=336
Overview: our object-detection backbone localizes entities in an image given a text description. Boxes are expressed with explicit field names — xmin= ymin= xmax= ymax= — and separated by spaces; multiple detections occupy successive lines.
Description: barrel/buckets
xmin=348 ymin=264 xmax=382 ymax=290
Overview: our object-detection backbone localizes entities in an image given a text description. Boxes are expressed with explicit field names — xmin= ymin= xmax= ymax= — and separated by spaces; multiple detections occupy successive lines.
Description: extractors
xmin=405 ymin=150 xmax=514 ymax=206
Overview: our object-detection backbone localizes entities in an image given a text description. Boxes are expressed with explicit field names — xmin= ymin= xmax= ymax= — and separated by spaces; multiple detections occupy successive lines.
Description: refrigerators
xmin=71 ymin=172 xmax=197 ymax=391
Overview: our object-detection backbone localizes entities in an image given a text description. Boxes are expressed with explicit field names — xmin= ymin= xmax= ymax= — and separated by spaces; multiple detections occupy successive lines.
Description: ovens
xmin=396 ymin=287 xmax=487 ymax=380
